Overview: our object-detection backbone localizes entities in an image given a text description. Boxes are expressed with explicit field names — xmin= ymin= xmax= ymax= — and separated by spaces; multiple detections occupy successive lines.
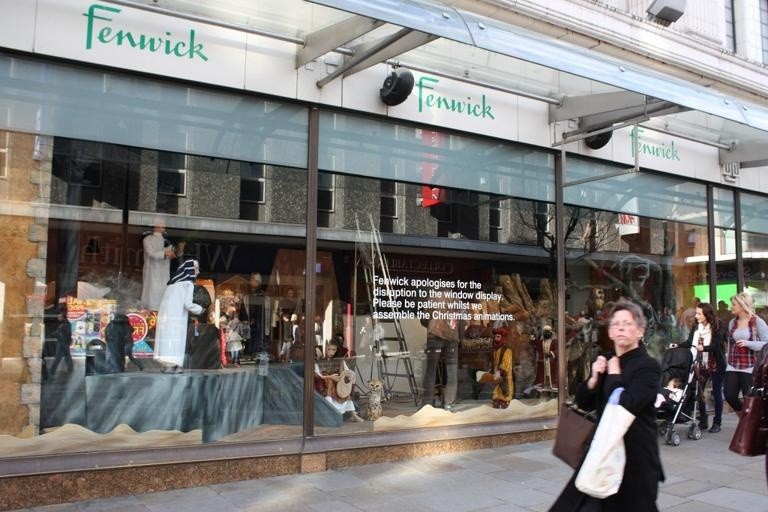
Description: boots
xmin=709 ymin=417 xmax=721 ymax=433
xmin=696 ymin=414 xmax=708 ymax=430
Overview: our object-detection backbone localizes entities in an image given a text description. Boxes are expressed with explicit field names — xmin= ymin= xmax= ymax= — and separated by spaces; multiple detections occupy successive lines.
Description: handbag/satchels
xmin=574 ymin=404 xmax=637 ymax=499
xmin=728 ymin=386 xmax=768 ymax=456
xmin=552 ymin=403 xmax=597 ymax=470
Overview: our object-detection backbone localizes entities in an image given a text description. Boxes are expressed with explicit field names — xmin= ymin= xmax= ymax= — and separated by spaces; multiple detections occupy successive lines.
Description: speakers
xmin=584 ymin=125 xmax=615 ymax=150
xmin=379 ymin=66 xmax=416 ymax=108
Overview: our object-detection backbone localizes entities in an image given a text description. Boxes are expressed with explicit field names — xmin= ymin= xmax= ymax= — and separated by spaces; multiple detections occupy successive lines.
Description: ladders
xmin=352 ymin=211 xmax=421 ymax=408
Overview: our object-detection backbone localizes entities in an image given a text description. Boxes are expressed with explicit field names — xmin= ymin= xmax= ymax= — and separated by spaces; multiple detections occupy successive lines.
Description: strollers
xmin=656 ymin=344 xmax=704 ymax=446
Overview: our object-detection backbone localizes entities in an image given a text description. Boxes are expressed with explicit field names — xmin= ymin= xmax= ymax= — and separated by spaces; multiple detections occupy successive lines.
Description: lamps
xmin=646 ymin=1 xmax=687 ymax=27
